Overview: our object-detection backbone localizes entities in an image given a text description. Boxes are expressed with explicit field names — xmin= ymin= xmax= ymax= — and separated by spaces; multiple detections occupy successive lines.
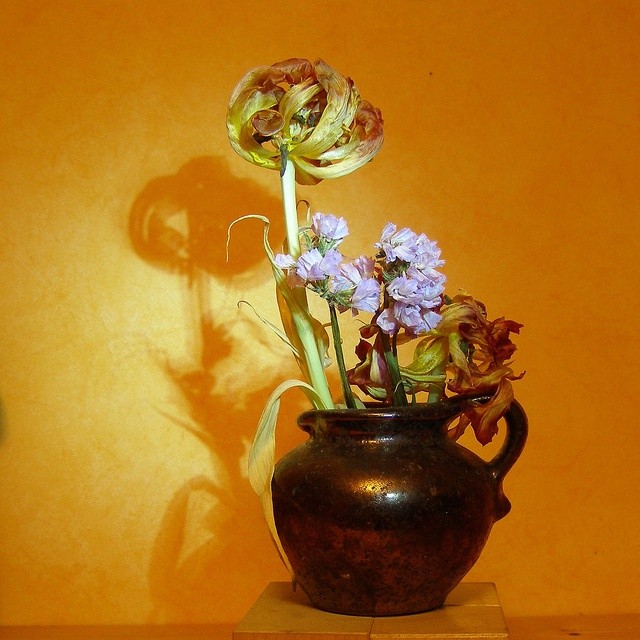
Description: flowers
xmin=226 ymin=57 xmax=526 ymax=591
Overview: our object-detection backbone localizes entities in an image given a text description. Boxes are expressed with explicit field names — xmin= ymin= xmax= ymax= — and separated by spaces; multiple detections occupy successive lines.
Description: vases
xmin=271 ymin=392 xmax=529 ymax=616
